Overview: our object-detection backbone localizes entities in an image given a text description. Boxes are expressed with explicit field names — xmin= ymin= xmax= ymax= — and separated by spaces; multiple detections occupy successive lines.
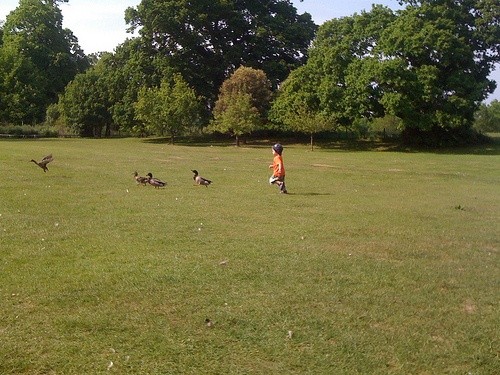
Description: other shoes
xmin=280 ymin=189 xmax=288 ymax=194
xmin=279 ymin=181 xmax=285 ymax=190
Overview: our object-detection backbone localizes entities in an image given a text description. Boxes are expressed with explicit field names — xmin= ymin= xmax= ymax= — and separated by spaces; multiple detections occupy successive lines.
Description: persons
xmin=269 ymin=144 xmax=287 ymax=194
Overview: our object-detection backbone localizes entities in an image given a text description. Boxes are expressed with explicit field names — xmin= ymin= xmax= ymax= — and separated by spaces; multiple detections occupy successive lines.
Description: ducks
xmin=133 ymin=171 xmax=166 ymax=189
xmin=30 ymin=153 xmax=54 ymax=172
xmin=190 ymin=169 xmax=212 ymax=189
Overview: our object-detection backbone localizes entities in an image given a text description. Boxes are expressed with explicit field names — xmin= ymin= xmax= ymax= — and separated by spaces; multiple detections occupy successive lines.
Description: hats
xmin=273 ymin=143 xmax=283 ymax=155
